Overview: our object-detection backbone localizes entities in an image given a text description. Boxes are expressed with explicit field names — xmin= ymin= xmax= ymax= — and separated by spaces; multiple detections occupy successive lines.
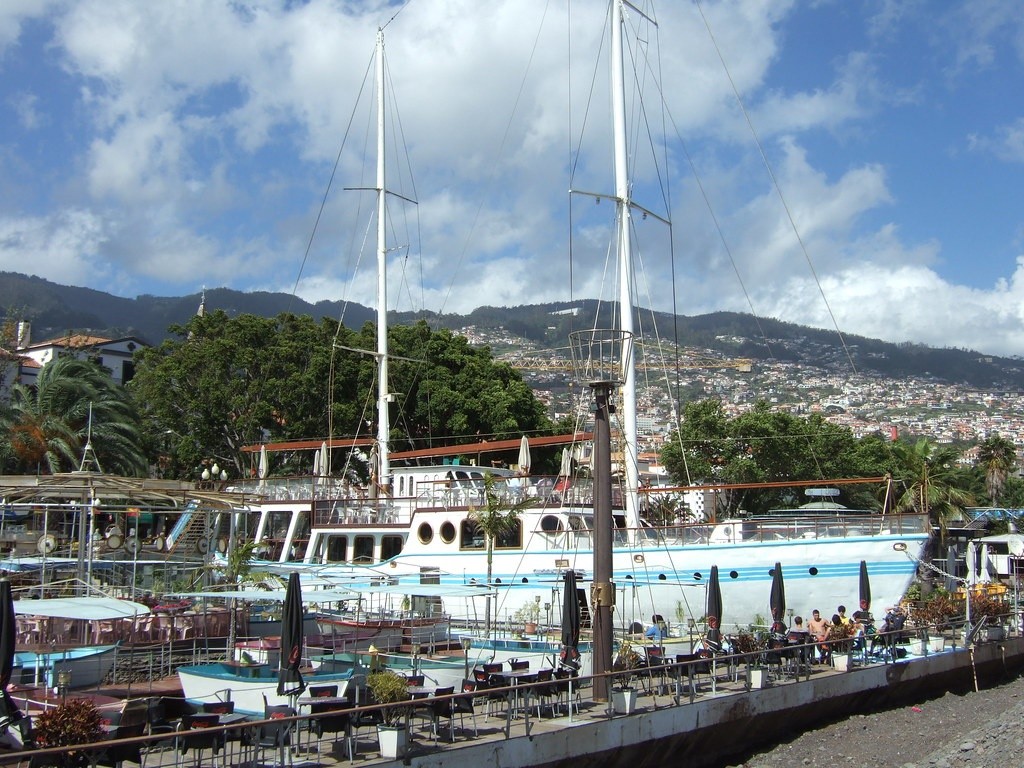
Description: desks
xmin=178 ymin=710 xmax=249 ymax=767
xmin=293 ymin=695 xmax=352 ymax=765
xmin=221 ymin=660 xmax=265 ymax=678
xmin=483 ymin=666 xmax=559 ymax=724
xmin=401 ymin=684 xmax=458 ymax=746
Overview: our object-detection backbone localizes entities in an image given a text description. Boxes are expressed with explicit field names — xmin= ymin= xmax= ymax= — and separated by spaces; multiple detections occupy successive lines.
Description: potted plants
xmin=607 ymin=643 xmax=644 ymax=714
xmin=694 ymin=613 xmax=708 ymax=632
xmin=983 ymin=602 xmax=1002 ymax=641
xmin=910 ymin=605 xmax=955 ymax=656
xmin=753 ymin=613 xmax=765 ymax=643
xmin=673 ymin=600 xmax=688 ymax=638
xmin=997 ymin=601 xmax=1015 ymax=639
xmin=958 ymin=605 xmax=977 ymax=645
xmin=365 ymin=670 xmax=417 ymax=759
xmin=827 ymin=625 xmax=857 ymax=672
xmin=513 ymin=598 xmax=540 ymax=636
xmin=736 ymin=620 xmax=775 ymax=690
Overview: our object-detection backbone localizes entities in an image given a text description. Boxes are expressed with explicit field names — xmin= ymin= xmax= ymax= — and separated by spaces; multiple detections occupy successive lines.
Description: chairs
xmin=0 ymin=613 xmax=909 ymax=767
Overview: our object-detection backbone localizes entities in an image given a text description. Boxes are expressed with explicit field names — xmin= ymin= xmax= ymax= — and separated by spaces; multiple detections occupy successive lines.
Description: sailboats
xmin=211 ymin=0 xmax=932 ymax=635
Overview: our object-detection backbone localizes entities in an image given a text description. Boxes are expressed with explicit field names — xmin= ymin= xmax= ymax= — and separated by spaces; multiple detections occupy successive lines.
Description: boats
xmin=175 ymin=582 xmax=707 ymax=721
xmin=9 ymin=597 xmax=151 ymax=692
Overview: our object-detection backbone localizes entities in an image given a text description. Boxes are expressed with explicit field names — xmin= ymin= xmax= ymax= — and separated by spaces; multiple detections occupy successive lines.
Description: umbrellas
xmin=317 ymin=441 xmax=331 ymax=485
xmin=313 ymin=449 xmax=320 ymax=476
xmin=559 ymin=446 xmax=572 ymax=476
xmin=704 ymin=562 xmax=724 ymax=695
xmin=857 ymin=558 xmax=871 ymax=664
xmin=945 ymin=544 xmax=957 ymax=610
xmin=556 ymin=568 xmax=582 ymax=722
xmin=518 ymin=435 xmax=531 ymax=475
xmin=258 ymin=443 xmax=270 ymax=493
xmin=0 ymin=570 xmax=23 ymax=739
xmin=967 ymin=540 xmax=979 ymax=587
xmin=980 ymin=543 xmax=992 ymax=582
xmin=278 ymin=570 xmax=305 ymax=765
xmin=771 ymin=559 xmax=786 ymax=680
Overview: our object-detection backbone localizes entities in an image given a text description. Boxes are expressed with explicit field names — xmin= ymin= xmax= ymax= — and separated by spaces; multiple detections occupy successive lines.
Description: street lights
xmin=199 ymin=463 xmax=229 ymax=560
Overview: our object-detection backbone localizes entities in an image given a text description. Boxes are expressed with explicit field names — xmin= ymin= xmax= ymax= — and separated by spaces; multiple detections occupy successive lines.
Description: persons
xmin=790 ymin=617 xmax=808 ymax=631
xmin=838 ymin=605 xmax=849 ymax=625
xmin=809 ymin=610 xmax=833 ymax=664
xmin=830 ymin=614 xmax=853 ymax=653
xmin=860 ymin=599 xmax=884 ymax=641
xmin=854 ymin=614 xmax=864 ymax=634
xmin=867 ymin=606 xmax=907 ymax=657
xmin=646 ymin=615 xmax=667 ymax=640
